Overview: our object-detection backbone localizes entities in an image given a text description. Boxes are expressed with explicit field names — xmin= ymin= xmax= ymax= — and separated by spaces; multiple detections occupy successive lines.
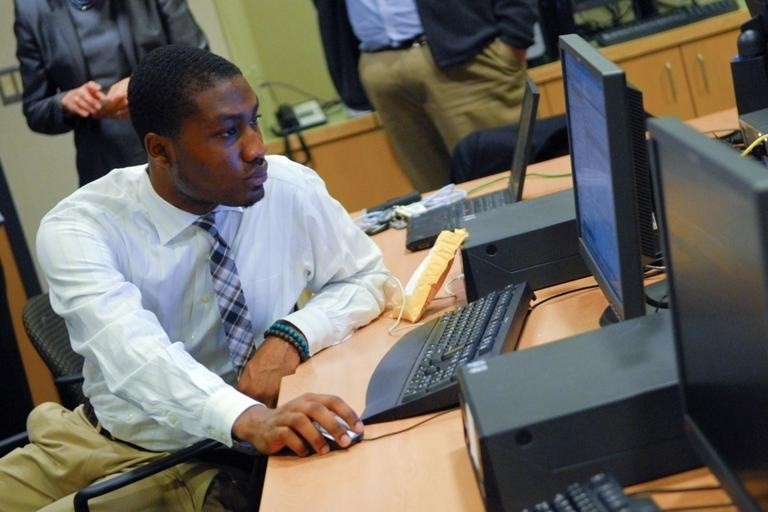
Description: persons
xmin=9 ymin=0 xmax=211 ymax=191
xmin=1 ymin=42 xmax=391 ymax=512
xmin=311 ymin=2 xmax=539 ymax=196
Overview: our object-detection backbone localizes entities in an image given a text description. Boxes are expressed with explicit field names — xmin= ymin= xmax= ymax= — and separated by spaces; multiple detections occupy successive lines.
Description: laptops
xmin=405 ymin=79 xmax=540 ymax=252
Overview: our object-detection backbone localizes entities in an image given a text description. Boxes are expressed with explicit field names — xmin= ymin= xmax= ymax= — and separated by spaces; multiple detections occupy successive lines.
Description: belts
xmin=83 ymin=399 xmax=167 ymax=453
xmin=358 ymin=32 xmax=428 ymax=51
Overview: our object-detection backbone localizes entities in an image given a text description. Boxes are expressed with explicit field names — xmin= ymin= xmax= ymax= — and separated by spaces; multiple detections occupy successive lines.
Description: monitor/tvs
xmin=558 ymin=33 xmax=670 ymax=326
xmin=644 ymin=115 xmax=767 ymax=512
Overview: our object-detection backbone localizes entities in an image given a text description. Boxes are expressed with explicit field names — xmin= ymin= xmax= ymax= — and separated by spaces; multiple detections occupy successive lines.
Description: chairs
xmin=0 ymin=286 xmax=260 ymax=512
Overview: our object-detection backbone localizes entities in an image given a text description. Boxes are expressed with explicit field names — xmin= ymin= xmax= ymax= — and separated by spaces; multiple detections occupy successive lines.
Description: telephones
xmin=269 ymin=98 xmax=328 ymax=138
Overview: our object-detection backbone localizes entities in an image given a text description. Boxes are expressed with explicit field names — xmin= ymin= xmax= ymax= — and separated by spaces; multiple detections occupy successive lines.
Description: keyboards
xmin=595 ymin=0 xmax=739 ymax=47
xmin=521 ymin=469 xmax=660 ymax=512
xmin=361 ymin=280 xmax=535 ymax=426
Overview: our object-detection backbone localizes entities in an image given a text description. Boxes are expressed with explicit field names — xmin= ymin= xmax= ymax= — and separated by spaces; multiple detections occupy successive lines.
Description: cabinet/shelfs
xmin=541 ymin=4 xmax=752 ymax=121
xmin=265 ymin=84 xmax=553 ymax=214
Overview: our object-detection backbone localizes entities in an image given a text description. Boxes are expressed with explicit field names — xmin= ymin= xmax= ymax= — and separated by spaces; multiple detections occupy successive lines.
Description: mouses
xmin=281 ymin=411 xmax=361 ymax=456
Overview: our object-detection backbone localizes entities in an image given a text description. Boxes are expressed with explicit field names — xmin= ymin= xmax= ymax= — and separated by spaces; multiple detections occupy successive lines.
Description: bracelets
xmin=271 ymin=322 xmax=309 ymax=357
xmin=263 ymin=329 xmax=306 ymax=362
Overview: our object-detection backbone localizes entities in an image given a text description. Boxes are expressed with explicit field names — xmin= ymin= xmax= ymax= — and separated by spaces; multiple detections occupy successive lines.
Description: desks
xmin=255 ymin=104 xmax=765 ymax=510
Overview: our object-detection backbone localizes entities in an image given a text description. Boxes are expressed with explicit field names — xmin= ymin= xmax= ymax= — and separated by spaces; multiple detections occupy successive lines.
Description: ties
xmin=193 ymin=211 xmax=257 ymax=386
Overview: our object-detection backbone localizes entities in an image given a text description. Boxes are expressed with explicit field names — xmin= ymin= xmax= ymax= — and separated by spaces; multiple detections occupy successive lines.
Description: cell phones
xmin=366 ymin=191 xmax=420 ymax=214
